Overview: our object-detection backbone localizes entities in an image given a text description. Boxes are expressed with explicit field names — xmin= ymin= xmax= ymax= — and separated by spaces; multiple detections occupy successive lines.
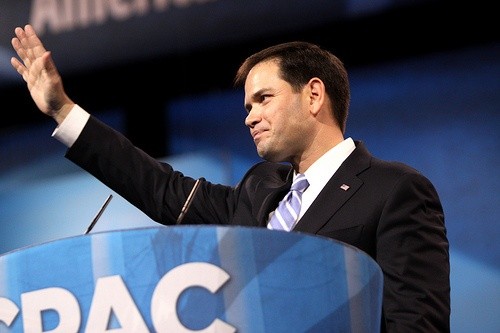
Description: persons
xmin=10 ymin=23 xmax=451 ymax=333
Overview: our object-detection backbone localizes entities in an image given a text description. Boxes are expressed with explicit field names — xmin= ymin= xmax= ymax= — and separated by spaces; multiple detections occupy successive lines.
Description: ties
xmin=267 ymin=174 xmax=310 ymax=231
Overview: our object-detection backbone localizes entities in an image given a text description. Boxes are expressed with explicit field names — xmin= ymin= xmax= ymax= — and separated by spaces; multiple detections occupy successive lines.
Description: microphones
xmin=176 ymin=179 xmax=202 ymax=225
xmin=84 ymin=195 xmax=113 ymax=234
